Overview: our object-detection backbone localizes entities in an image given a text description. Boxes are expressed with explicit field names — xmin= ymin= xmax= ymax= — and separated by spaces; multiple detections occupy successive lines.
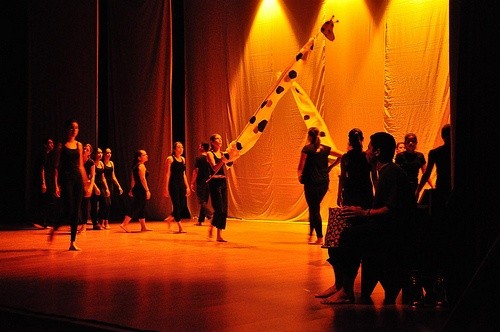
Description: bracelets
xmin=367 ymin=209 xmax=371 ymax=216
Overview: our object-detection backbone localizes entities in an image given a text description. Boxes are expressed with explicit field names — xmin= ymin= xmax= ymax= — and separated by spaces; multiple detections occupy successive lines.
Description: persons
xmin=337 ymin=128 xmax=379 ymax=209
xmin=164 ymin=142 xmax=191 ymax=233
xmin=34 ymin=138 xmax=54 ymax=229
xmin=77 ymin=144 xmax=96 ymax=232
xmin=297 ymin=127 xmax=343 ymax=246
xmin=100 ymin=146 xmax=123 ymax=229
xmin=206 ymin=133 xmax=229 ymax=243
xmin=48 ymin=121 xmax=88 ymax=251
xmin=190 ymin=142 xmax=214 ymax=226
xmin=89 ymin=147 xmax=110 ymax=230
xmin=396 ymin=141 xmax=407 ymax=154
xmin=395 ymin=133 xmax=435 ymax=191
xmin=120 ymin=149 xmax=153 ymax=233
xmin=313 ymin=132 xmax=416 ymax=304
xmin=415 ymin=124 xmax=450 ymax=201
